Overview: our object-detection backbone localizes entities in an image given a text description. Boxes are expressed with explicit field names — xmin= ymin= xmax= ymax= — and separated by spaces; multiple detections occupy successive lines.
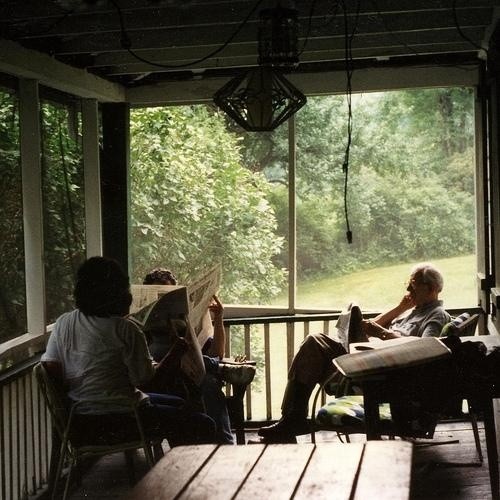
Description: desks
xmin=124 ymin=441 xmax=414 ymax=500
xmin=348 ymin=339 xmax=500 ymax=500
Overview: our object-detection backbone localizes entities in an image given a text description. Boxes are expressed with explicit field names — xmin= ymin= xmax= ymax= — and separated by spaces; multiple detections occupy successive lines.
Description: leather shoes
xmin=247 ymin=416 xmax=310 ymax=444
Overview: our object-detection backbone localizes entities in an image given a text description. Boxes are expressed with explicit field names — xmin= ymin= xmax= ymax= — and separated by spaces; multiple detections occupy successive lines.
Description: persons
xmin=140 ymin=267 xmax=257 ymax=444
xmin=257 ymin=263 xmax=452 ymax=439
xmin=39 ymin=254 xmax=216 ymax=450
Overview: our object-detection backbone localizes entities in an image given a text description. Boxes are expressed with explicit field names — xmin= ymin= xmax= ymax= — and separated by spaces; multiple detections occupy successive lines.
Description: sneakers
xmin=216 ymin=364 xmax=256 ymax=385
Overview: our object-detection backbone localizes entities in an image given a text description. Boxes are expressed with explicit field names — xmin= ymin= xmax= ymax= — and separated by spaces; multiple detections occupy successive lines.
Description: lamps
xmin=212 ymin=68 xmax=308 ymax=131
xmin=258 ymin=9 xmax=301 ymax=71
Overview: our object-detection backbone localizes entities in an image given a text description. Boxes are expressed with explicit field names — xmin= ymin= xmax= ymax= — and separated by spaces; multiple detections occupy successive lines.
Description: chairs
xmin=33 ymin=357 xmax=256 ymax=500
xmin=312 ymin=313 xmax=483 ymax=463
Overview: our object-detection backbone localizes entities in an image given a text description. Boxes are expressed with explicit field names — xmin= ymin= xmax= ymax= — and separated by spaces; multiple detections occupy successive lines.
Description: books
xmin=332 ymin=337 xmax=452 ymax=378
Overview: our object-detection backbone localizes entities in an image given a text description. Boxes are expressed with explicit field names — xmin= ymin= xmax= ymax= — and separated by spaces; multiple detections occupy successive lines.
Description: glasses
xmin=403 ymin=282 xmax=424 ymax=287
